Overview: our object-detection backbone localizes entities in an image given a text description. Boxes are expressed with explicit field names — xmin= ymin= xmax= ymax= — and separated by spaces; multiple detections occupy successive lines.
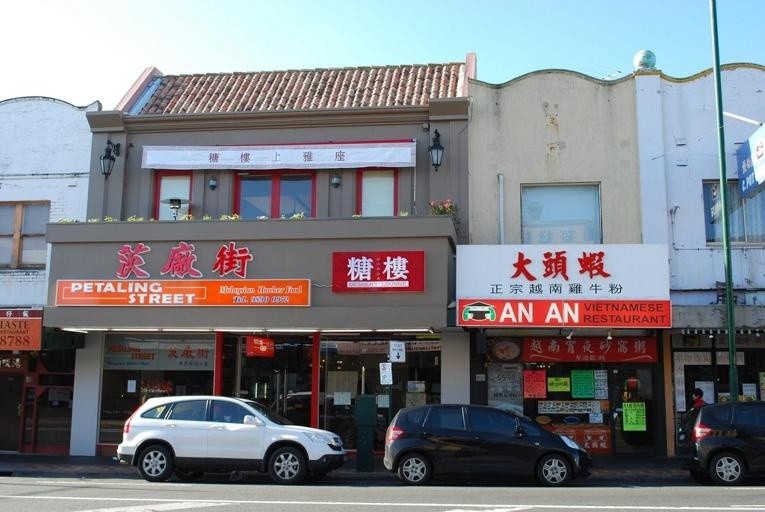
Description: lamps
xmin=209 ymin=176 xmax=218 ymax=190
xmin=330 ymin=173 xmax=340 ymax=188
xmin=427 ymin=128 xmax=445 ymax=173
xmin=100 ymin=139 xmax=120 ymax=182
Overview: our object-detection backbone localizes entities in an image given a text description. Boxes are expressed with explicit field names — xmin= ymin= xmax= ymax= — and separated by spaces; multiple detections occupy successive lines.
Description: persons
xmin=691 ymin=387 xmax=707 ymax=410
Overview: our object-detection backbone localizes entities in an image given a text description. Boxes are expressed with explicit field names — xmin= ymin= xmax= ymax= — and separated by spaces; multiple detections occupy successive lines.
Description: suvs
xmin=273 ymin=391 xmax=387 ymax=444
xmin=111 ymin=395 xmax=346 ymax=485
xmin=384 ymin=405 xmax=592 ymax=486
xmin=677 ymin=401 xmax=764 ymax=484
